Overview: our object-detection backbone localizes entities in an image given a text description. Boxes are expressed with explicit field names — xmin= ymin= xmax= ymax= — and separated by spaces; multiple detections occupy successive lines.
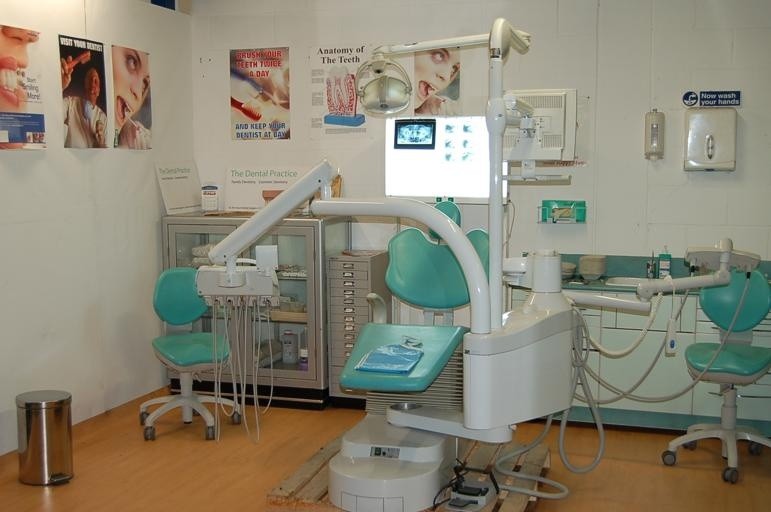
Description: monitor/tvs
xmin=384 ymin=116 xmax=508 ymax=206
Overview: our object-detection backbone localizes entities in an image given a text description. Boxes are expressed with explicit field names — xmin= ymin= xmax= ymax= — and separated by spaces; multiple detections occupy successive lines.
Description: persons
xmin=63 ymin=52 xmax=107 ymax=147
xmin=1 ymin=25 xmax=40 ymax=111
xmin=415 ymin=48 xmax=461 ymax=116
xmin=113 ymin=47 xmax=151 ymax=150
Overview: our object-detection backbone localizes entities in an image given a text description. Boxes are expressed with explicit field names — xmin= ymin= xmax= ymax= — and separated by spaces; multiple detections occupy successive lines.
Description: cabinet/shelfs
xmin=327 ymin=248 xmax=390 ymax=407
xmin=166 ymin=215 xmax=352 ymax=407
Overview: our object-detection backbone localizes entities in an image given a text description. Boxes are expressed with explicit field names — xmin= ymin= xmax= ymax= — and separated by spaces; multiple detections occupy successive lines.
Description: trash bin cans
xmin=16 ymin=390 xmax=74 ymax=486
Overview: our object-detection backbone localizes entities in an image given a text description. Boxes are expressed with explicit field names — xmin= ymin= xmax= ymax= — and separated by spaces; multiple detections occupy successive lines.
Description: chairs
xmin=660 ymin=268 xmax=771 ymax=486
xmin=141 ymin=267 xmax=244 ymax=443
xmin=338 ymin=199 xmax=501 ymax=394
